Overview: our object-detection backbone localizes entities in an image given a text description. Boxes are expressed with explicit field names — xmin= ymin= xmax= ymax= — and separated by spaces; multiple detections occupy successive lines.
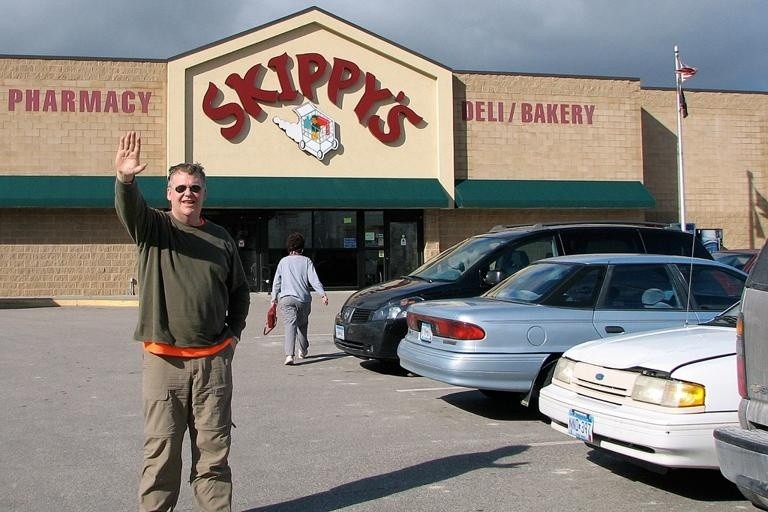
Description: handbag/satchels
xmin=267 ymin=303 xmax=277 ymax=328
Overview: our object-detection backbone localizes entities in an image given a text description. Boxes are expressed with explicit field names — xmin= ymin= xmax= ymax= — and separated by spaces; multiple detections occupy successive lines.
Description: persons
xmin=114 ymin=131 xmax=250 ymax=512
xmin=271 ymin=233 xmax=328 ymax=365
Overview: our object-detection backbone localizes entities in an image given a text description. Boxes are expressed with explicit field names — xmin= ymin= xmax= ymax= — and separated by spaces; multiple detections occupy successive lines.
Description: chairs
xmin=510 ymin=251 xmax=529 ymax=269
xmin=642 ymin=288 xmax=671 ymax=308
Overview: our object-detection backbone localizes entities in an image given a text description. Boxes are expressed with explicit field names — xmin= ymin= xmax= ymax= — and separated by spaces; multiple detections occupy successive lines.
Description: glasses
xmin=170 ymin=185 xmax=205 ymax=193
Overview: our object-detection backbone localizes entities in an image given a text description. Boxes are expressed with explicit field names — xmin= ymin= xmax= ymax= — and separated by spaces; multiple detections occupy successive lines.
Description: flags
xmin=675 ymin=53 xmax=697 ymax=118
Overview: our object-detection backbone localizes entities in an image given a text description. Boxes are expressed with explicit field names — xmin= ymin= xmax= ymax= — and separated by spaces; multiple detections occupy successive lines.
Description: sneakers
xmin=299 ymin=350 xmax=308 ymax=359
xmin=284 ymin=356 xmax=294 ymax=365
